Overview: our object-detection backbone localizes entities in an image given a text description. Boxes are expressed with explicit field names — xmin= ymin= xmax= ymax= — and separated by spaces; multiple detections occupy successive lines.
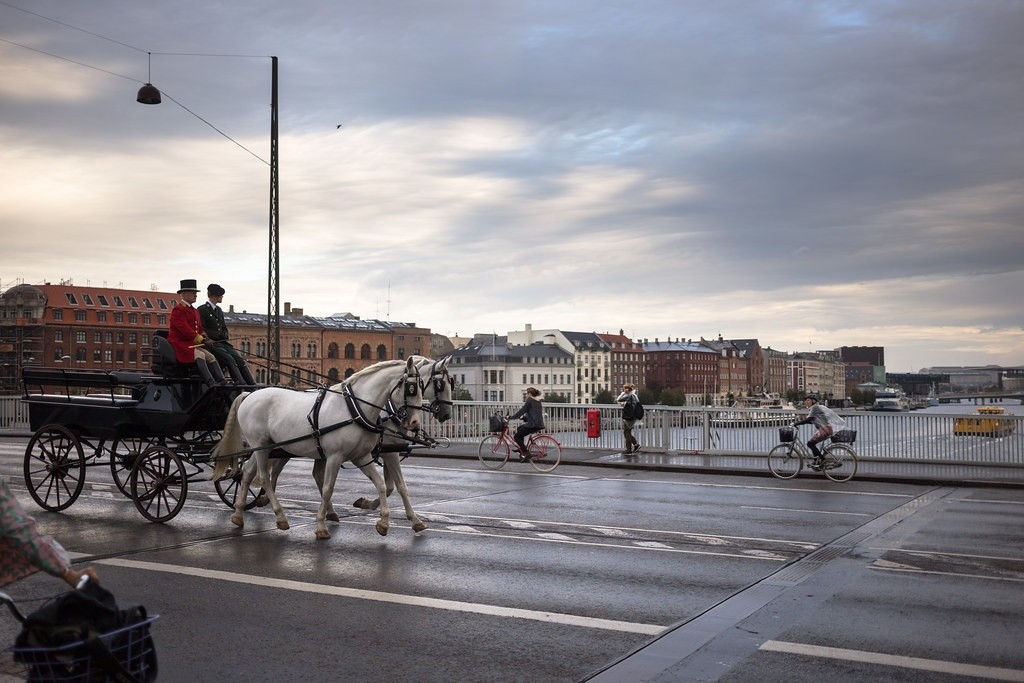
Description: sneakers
xmin=633 ymin=444 xmax=641 ymax=453
xmin=623 ymin=450 xmax=632 ymax=456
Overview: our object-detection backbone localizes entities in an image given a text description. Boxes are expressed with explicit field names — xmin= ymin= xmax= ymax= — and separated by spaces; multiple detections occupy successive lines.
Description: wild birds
xmin=337 ymin=125 xmax=341 ymax=129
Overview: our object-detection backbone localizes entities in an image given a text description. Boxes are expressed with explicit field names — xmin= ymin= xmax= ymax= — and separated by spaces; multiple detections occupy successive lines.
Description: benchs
xmin=22 ymin=365 xmax=153 ymax=407
xmin=150 ymin=329 xmax=198 ymax=377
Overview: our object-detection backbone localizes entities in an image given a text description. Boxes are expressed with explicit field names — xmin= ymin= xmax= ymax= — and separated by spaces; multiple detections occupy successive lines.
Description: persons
xmin=167 ymin=279 xmax=227 ymax=387
xmin=617 ymin=382 xmax=641 ymax=455
xmin=505 ymin=388 xmax=545 ymax=462
xmin=0 ymin=473 xmax=100 ymax=591
xmin=790 ymin=394 xmax=833 ymax=468
xmin=197 ymin=285 xmax=257 ymax=391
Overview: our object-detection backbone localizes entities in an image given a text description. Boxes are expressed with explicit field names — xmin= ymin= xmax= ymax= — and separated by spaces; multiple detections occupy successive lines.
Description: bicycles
xmin=767 ymin=425 xmax=858 ymax=483
xmin=477 ymin=416 xmax=562 ymax=473
xmin=0 ymin=573 xmax=160 ymax=683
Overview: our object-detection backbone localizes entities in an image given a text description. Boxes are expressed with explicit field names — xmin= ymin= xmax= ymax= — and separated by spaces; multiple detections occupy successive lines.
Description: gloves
xmin=197 ymin=335 xmax=216 ymax=349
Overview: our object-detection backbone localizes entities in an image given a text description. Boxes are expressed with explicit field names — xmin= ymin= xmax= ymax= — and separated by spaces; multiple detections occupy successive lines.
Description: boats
xmin=701 ymin=386 xmax=797 ymax=428
xmin=953 ymin=407 xmax=1015 ymax=438
xmin=873 ymin=380 xmax=941 ymax=411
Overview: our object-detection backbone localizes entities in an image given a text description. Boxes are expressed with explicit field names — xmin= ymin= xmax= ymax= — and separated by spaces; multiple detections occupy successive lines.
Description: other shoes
xmin=512 ymin=448 xmax=521 ymax=453
xmin=807 ymin=461 xmax=816 ymax=466
xmin=819 ymin=458 xmax=827 ymax=467
xmin=521 ymin=453 xmax=532 ymax=462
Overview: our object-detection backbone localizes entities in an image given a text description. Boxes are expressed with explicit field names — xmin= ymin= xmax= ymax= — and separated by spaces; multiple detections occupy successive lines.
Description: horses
xmin=211 ymin=354 xmax=453 ymax=538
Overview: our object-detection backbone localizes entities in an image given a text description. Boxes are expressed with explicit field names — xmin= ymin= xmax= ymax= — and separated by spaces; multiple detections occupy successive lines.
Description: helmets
xmin=804 ymin=393 xmax=818 ymax=403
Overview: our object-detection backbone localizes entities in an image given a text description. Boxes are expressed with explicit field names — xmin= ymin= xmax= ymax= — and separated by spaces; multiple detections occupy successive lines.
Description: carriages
xmin=22 ymin=354 xmax=455 ymax=540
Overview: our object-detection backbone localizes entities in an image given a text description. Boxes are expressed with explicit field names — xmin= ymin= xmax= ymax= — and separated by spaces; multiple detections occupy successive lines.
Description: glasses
xmin=527 ymin=392 xmax=530 ymax=394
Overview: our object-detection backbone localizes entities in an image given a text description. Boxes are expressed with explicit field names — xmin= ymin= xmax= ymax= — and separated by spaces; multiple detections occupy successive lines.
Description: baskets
xmin=778 ymin=428 xmax=793 ymax=442
xmin=831 ymin=430 xmax=857 ymax=443
xmin=490 ymin=417 xmax=504 ymax=432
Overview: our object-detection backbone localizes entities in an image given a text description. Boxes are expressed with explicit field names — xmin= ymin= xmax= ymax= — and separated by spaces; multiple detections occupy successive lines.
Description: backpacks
xmin=632 ymin=395 xmax=644 ymax=420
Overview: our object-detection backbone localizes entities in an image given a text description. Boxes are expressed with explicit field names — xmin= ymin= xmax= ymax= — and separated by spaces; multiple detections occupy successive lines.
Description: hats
xmin=177 ymin=279 xmax=201 ymax=294
xmin=207 ymin=284 xmax=225 ymax=295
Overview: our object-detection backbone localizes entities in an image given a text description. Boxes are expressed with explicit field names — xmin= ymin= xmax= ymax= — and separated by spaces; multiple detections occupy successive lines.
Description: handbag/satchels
xmin=622 ymin=397 xmax=634 ymax=421
xmin=14 ymin=578 xmax=157 ymax=683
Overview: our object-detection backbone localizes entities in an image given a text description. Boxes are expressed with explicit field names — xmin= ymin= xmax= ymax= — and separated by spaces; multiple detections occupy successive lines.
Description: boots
xmin=195 ymin=357 xmax=233 ymax=388
xmin=226 ymin=360 xmax=256 ymax=385
